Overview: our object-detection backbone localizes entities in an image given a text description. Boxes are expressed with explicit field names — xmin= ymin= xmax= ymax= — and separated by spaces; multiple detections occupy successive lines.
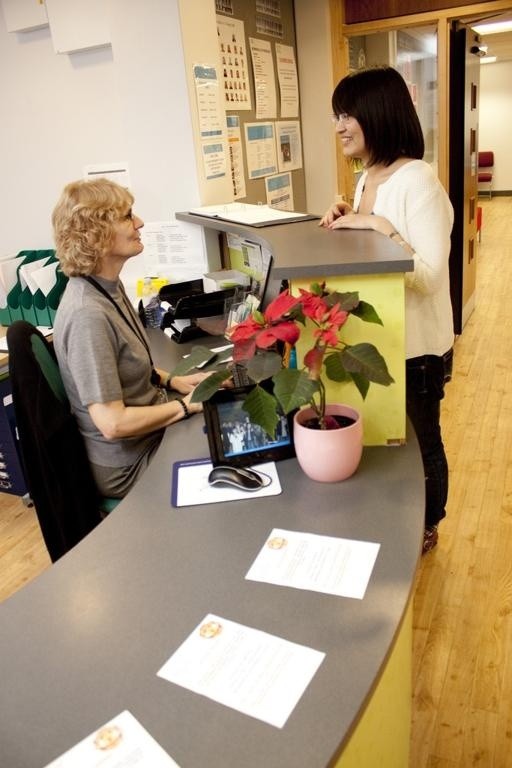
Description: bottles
xmin=141 ymin=277 xmax=165 ymax=329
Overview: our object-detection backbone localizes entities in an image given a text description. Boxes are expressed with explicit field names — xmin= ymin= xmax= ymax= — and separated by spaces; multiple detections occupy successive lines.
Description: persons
xmin=221 ymin=415 xmax=288 ymax=452
xmin=317 ymin=63 xmax=455 ymax=556
xmin=51 ymin=178 xmax=234 ymax=498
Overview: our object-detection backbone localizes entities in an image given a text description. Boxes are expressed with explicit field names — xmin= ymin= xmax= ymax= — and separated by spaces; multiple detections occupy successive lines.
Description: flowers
xmin=163 ymin=275 xmax=397 ymax=445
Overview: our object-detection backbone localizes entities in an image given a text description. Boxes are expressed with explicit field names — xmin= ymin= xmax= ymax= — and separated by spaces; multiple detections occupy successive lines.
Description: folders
xmin=189 ymin=202 xmax=322 ymax=228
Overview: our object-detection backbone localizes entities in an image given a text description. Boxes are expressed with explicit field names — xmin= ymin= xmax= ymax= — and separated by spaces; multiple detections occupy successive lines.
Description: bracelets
xmin=388 ymin=230 xmax=398 ymax=236
xmin=166 ymin=371 xmax=174 ymax=390
xmin=399 ymin=240 xmax=407 ymax=245
xmin=175 ymin=397 xmax=190 ymax=419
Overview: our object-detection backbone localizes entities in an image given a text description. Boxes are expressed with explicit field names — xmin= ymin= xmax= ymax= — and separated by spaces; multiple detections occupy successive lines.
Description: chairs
xmin=6 ymin=321 xmax=103 ymax=564
xmin=478 ymin=152 xmax=494 ymax=200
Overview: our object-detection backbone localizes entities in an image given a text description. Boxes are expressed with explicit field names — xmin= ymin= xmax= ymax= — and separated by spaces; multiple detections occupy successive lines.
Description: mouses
xmin=207 ymin=466 xmax=264 ymax=492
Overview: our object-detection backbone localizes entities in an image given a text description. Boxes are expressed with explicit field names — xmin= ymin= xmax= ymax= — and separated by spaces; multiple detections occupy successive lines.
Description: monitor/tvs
xmin=258 ymin=256 xmax=289 ymax=314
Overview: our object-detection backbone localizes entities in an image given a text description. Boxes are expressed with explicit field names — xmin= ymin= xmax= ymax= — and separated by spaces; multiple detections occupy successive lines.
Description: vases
xmin=292 ymin=405 xmax=364 ymax=482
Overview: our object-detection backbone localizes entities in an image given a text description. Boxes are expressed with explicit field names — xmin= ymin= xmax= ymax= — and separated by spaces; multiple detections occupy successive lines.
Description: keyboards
xmin=230 ymin=364 xmax=250 ymax=387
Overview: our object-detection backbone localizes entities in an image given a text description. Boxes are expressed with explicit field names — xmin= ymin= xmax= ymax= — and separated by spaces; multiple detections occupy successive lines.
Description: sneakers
xmin=422 ymin=526 xmax=438 ymax=551
